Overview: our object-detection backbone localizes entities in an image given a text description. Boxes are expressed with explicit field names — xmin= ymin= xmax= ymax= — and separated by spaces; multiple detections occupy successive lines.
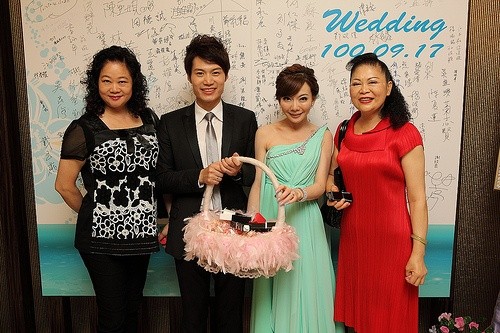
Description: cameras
xmin=330 ymin=191 xmax=353 ymax=202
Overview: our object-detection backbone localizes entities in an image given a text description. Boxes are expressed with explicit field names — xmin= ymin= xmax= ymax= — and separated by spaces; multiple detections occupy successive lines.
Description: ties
xmin=205 ymin=112 xmax=222 ymax=211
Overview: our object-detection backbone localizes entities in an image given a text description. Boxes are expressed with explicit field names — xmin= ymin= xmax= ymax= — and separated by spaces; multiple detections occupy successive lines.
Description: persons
xmin=158 ymin=33 xmax=258 ymax=333
xmin=55 ymin=47 xmax=159 ymax=333
xmin=325 ymin=52 xmax=428 ymax=333
xmin=245 ymin=64 xmax=348 ymax=333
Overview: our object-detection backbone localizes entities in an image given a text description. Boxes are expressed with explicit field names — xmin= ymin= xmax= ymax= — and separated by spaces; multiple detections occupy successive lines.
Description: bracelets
xmin=295 ymin=187 xmax=307 ymax=203
xmin=410 ymin=232 xmax=426 ymax=245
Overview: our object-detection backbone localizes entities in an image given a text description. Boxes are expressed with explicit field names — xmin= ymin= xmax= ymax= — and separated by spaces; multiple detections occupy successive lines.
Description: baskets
xmin=184 ymin=157 xmax=300 ymax=279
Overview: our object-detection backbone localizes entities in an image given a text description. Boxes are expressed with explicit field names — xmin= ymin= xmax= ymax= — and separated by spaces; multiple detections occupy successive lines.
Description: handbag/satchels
xmin=321 ymin=120 xmax=350 ymax=229
